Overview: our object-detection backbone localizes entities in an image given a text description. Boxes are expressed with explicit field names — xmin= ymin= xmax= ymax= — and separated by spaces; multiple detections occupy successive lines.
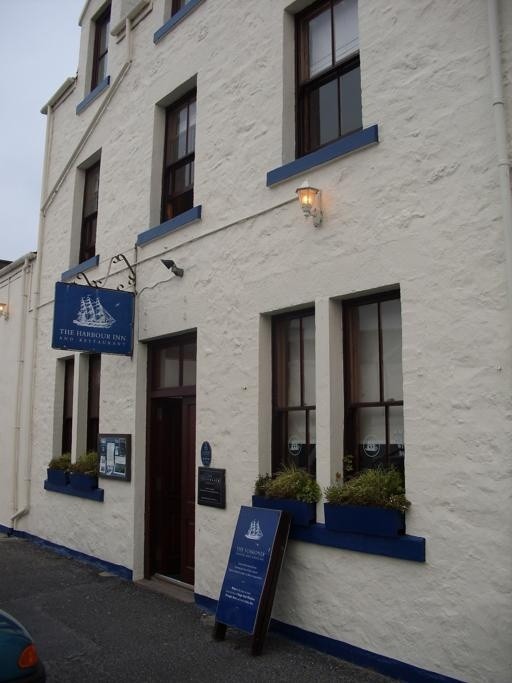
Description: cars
xmin=0 ymin=609 xmax=46 ymax=682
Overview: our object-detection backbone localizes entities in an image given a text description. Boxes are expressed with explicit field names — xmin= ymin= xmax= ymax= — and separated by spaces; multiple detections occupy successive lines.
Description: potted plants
xmin=47 ymin=452 xmax=69 ymax=484
xmin=252 ymin=463 xmax=321 ymax=527
xmin=69 ymin=451 xmax=100 ymax=491
xmin=325 ymin=461 xmax=410 ymax=533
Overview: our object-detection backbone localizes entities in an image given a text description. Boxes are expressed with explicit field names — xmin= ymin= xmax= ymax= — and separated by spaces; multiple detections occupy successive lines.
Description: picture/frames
xmin=98 ymin=433 xmax=130 ymax=481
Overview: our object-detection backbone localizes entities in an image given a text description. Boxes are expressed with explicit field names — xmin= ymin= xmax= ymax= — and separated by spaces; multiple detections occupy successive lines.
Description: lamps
xmin=295 ymin=175 xmax=324 ymax=230
